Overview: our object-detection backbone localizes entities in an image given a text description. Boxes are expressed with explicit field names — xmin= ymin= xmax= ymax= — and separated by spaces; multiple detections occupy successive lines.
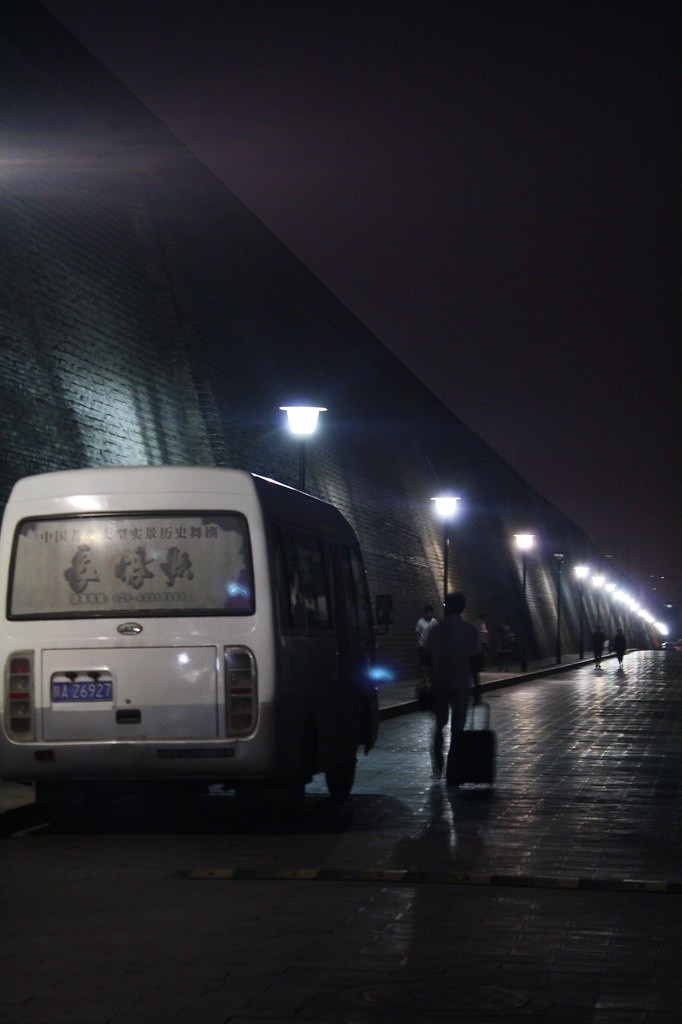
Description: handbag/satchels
xmin=416 ymin=681 xmax=433 ymax=712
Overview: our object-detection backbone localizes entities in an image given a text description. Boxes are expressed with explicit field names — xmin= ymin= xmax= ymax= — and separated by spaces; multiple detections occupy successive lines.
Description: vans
xmin=0 ymin=467 xmax=395 ymax=820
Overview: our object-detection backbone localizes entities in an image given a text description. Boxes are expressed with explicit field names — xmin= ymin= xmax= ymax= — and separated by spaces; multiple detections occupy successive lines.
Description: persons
xmin=477 ymin=620 xmax=512 ymax=671
xmin=423 ymin=592 xmax=482 ymax=786
xmin=417 ymin=605 xmax=439 ymax=681
xmin=614 ymin=629 xmax=627 ymax=663
xmin=590 ymin=625 xmax=605 ymax=668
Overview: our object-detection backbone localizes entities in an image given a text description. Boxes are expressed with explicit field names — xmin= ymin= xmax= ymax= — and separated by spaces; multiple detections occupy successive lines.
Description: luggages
xmin=460 ymin=701 xmax=496 ymax=785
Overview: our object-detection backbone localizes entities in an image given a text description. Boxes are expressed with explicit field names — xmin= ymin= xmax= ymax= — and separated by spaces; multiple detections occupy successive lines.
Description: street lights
xmin=278 ymin=402 xmax=330 ymax=494
xmin=429 ymin=489 xmax=464 ymax=603
xmin=511 ymin=530 xmax=542 ymax=675
xmin=553 ymin=550 xmax=668 ymax=666
